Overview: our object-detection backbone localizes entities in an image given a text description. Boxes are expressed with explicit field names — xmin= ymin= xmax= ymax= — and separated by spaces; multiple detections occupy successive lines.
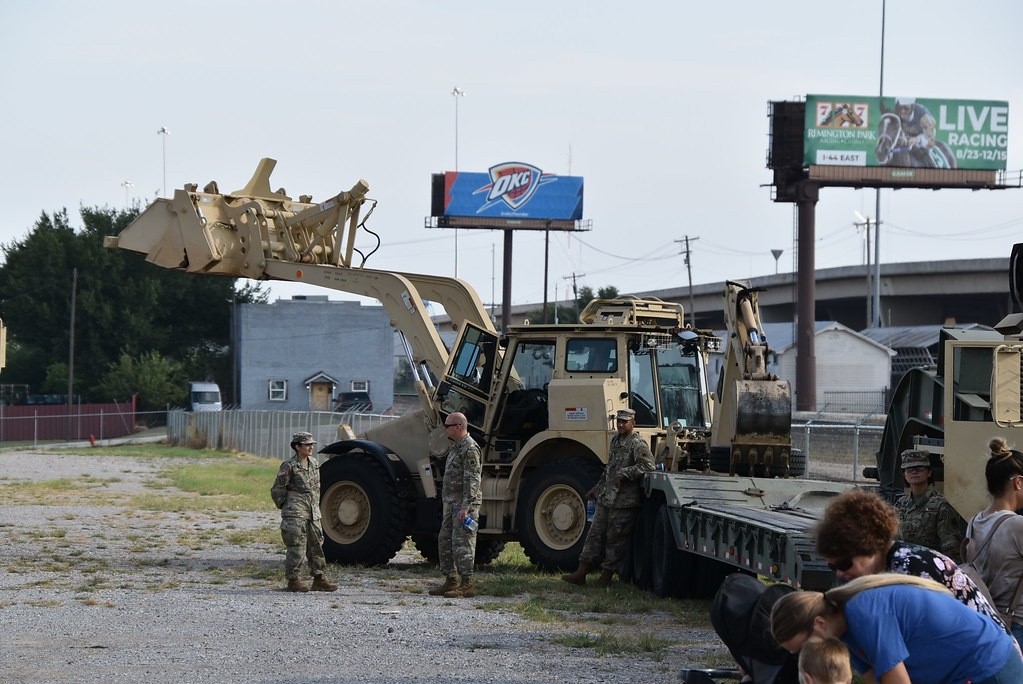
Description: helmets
xmin=895 ymin=97 xmax=915 ymax=105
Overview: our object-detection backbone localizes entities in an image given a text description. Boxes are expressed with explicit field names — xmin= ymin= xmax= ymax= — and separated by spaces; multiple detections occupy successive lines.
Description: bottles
xmin=453 ymin=508 xmax=478 ymax=531
xmin=586 ymin=495 xmax=595 ymax=522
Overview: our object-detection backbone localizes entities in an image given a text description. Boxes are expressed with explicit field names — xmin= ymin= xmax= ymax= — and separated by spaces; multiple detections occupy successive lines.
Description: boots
xmin=444 ymin=575 xmax=476 ymax=598
xmin=310 ymin=575 xmax=338 ymax=591
xmin=429 ymin=574 xmax=459 ymax=595
xmin=561 ymin=560 xmax=590 ymax=586
xmin=288 ymin=577 xmax=308 ymax=592
xmin=590 ymin=568 xmax=615 ymax=589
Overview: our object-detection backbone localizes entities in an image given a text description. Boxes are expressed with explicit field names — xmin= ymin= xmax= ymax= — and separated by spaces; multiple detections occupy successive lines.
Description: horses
xmin=874 ymin=98 xmax=957 ymax=168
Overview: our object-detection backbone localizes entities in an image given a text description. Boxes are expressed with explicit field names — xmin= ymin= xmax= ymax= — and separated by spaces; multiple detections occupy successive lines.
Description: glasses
xmin=444 ymin=424 xmax=461 ymax=429
xmin=828 ymin=548 xmax=854 ymax=571
xmin=906 ymin=466 xmax=928 ymax=472
xmin=790 ymin=620 xmax=813 ymax=656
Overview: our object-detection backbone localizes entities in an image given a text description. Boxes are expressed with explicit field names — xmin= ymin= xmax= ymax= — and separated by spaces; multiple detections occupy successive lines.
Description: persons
xmin=428 ymin=413 xmax=482 ymax=598
xmin=769 ymin=438 xmax=1023 ymax=684
xmin=562 ymin=409 xmax=655 ymax=586
xmin=270 ymin=432 xmax=338 ymax=592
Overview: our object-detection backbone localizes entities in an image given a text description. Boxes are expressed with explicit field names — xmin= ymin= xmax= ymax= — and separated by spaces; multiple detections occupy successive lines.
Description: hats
xmin=614 ymin=409 xmax=636 ymax=420
xmin=292 ymin=431 xmax=317 ymax=444
xmin=900 ymin=450 xmax=930 ymax=468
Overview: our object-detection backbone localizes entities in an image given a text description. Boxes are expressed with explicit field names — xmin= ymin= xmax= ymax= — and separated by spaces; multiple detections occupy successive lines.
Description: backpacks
xmin=957 ymin=514 xmax=1023 ymax=630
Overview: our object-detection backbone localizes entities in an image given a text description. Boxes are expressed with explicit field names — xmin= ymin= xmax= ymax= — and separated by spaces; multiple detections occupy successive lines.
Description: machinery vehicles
xmin=103 ymin=157 xmax=805 ymax=599
xmin=643 ymin=244 xmax=1023 ymax=594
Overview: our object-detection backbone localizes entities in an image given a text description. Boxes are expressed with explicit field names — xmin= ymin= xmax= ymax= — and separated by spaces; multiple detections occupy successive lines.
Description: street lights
xmin=453 ymin=87 xmax=464 ymax=276
xmin=157 ymin=127 xmax=170 ymax=198
xmin=121 ymin=180 xmax=133 ymax=210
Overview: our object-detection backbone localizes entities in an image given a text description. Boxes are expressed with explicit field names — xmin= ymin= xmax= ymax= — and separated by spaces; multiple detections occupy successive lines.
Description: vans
xmin=187 ymin=381 xmax=222 ymax=412
